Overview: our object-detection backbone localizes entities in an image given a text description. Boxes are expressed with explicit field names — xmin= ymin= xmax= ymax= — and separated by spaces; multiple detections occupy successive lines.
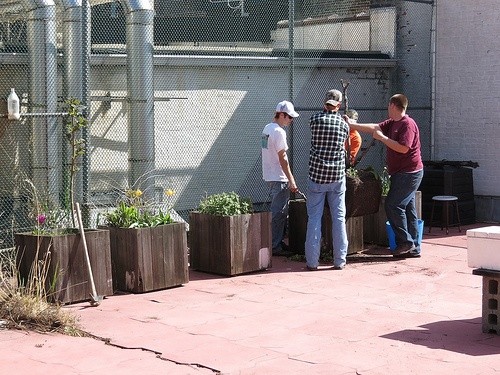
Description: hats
xmin=274 ymin=100 xmax=300 ymax=118
xmin=323 ymin=89 xmax=342 ymax=107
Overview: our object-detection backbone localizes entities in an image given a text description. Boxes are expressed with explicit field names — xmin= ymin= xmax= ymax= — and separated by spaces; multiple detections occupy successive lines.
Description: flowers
xmin=34 ymin=214 xmax=47 ymax=235
xmin=106 ymin=188 xmax=178 ymax=229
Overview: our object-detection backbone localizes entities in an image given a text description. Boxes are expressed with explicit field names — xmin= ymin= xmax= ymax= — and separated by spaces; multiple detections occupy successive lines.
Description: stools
xmin=429 ymin=196 xmax=461 ymax=234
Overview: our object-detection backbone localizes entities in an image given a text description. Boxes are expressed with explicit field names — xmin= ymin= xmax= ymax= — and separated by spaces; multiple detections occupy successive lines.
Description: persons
xmin=343 ymin=93 xmax=424 ymax=258
xmin=305 ymin=89 xmax=350 ymax=270
xmin=344 ymin=109 xmax=362 ymax=170
xmin=262 ymin=101 xmax=299 ymax=255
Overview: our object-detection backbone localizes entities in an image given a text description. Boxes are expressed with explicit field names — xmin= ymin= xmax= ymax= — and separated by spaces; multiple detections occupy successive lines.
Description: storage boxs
xmin=466 ymin=226 xmax=500 ymax=271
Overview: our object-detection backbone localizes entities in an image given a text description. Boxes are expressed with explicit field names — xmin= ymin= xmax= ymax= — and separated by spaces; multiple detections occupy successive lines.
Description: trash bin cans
xmin=424 ymin=159 xmax=474 ymax=226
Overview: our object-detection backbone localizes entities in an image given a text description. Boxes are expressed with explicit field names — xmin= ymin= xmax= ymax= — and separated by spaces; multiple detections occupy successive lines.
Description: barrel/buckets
xmin=386 ymin=219 xmax=424 ymax=254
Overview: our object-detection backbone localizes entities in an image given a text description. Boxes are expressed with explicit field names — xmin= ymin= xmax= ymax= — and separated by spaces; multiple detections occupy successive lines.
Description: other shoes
xmin=393 ymin=240 xmax=421 ymax=258
xmin=334 ymin=266 xmax=345 ymax=270
xmin=307 ymin=266 xmax=318 ymax=272
xmin=272 ymin=241 xmax=297 ymax=256
